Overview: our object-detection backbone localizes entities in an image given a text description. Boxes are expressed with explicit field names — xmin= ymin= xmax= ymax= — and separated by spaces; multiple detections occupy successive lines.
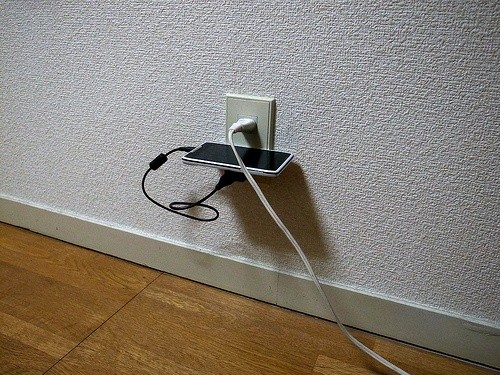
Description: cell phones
xmin=182 ymin=142 xmax=295 ymax=177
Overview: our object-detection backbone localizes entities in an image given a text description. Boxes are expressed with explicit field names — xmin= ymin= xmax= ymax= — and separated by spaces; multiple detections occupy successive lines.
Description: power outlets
xmin=221 ymin=93 xmax=275 ymax=156
